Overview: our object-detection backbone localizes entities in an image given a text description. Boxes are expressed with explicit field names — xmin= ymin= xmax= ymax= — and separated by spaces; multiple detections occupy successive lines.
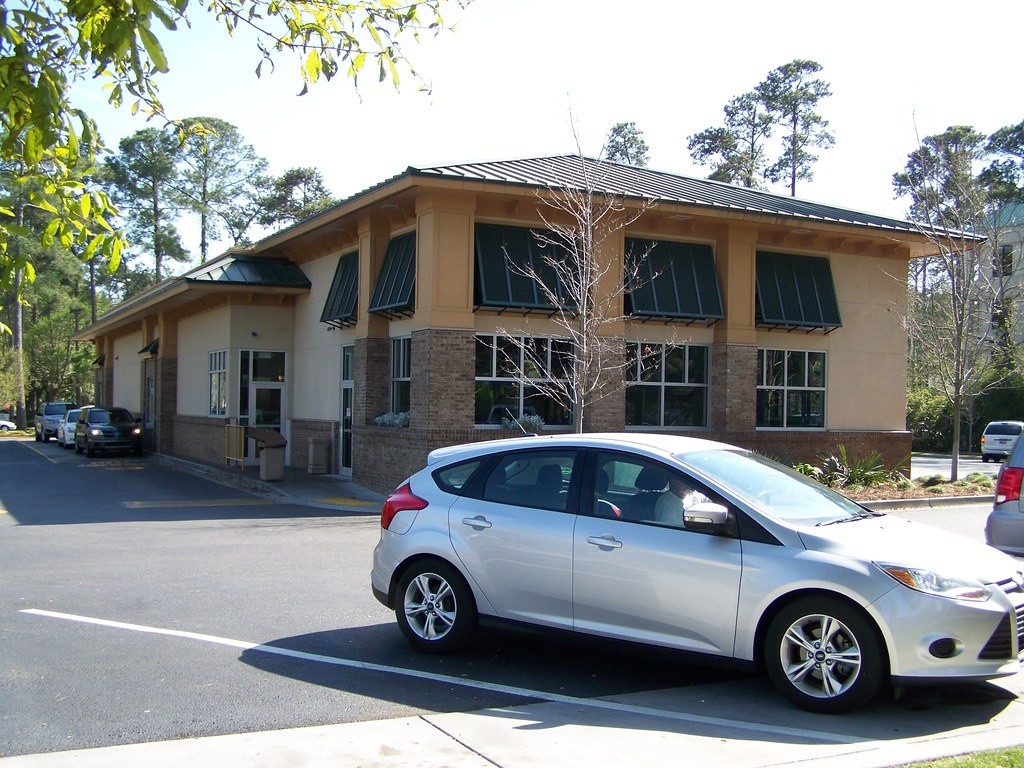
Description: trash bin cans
xmin=258 ymin=440 xmax=286 ymax=482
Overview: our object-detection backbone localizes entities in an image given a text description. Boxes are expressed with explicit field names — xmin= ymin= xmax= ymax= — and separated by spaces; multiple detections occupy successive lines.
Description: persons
xmin=654 ymin=474 xmax=715 ymax=527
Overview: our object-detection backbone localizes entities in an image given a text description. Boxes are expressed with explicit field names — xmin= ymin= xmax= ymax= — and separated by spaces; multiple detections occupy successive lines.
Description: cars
xmin=57 ymin=409 xmax=83 ymax=449
xmin=0 ymin=419 xmax=17 ymax=432
xmin=983 ymin=422 xmax=1024 ymax=556
xmin=371 ymin=433 xmax=1024 ymax=716
xmin=73 ymin=407 xmax=145 ymax=457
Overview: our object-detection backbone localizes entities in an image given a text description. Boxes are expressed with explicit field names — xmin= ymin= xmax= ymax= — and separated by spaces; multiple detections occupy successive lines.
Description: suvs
xmin=979 ymin=421 xmax=1024 ymax=463
xmin=34 ymin=402 xmax=79 ymax=443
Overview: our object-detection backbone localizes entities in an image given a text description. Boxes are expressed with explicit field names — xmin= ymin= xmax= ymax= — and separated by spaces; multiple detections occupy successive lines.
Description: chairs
xmin=594 ymin=464 xmax=622 ymax=518
xmin=527 ymin=464 xmax=567 ymax=508
xmin=484 ymin=466 xmax=506 ymax=501
xmin=628 ymin=467 xmax=669 ymax=519
